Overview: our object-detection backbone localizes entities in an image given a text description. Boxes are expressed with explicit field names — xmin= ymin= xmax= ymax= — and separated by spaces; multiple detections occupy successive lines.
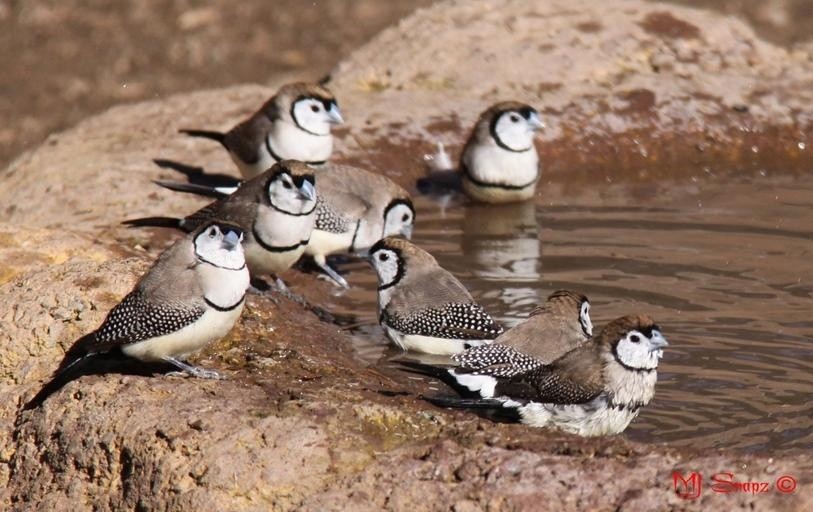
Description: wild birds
xmin=118 ymin=158 xmax=321 ymax=295
xmin=348 ymin=236 xmax=504 ymax=358
xmin=390 ymin=287 xmax=674 ymax=460
xmin=152 ymin=162 xmax=416 ymax=291
xmin=177 ymin=80 xmax=346 ymax=183
xmin=456 ymin=99 xmax=548 ymax=207
xmin=43 ymin=219 xmax=253 ymax=379
